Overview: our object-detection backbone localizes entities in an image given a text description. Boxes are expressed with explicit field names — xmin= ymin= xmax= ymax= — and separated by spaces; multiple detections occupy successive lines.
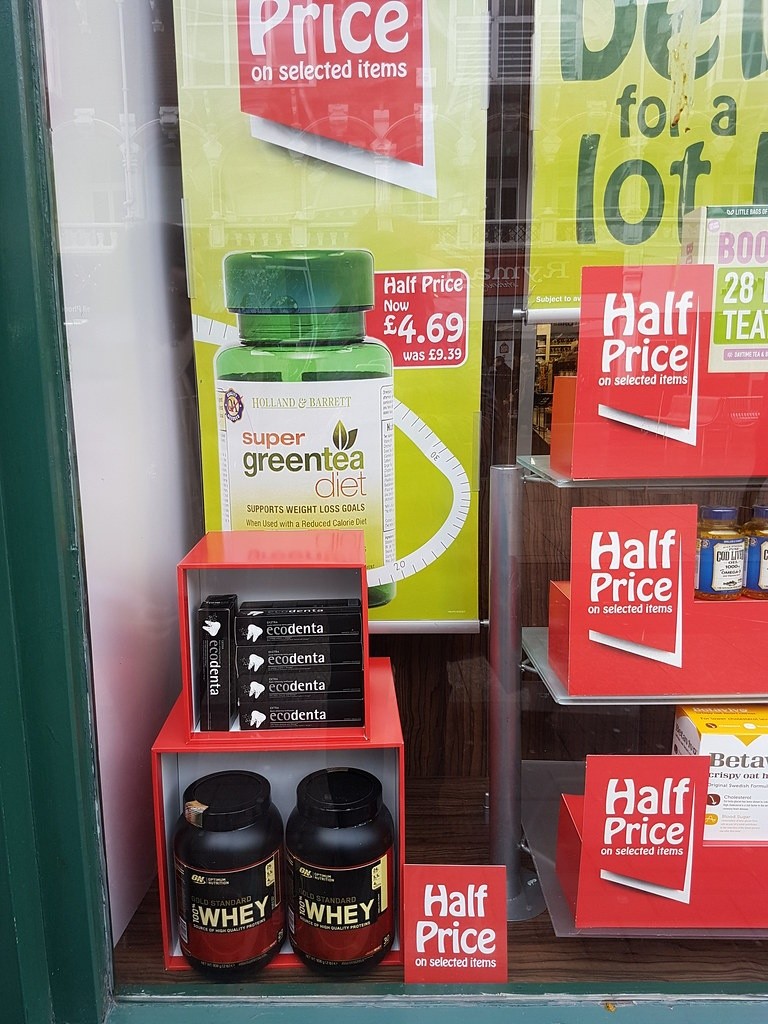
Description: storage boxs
xmin=196 ymin=593 xmax=239 ymax=731
xmin=547 ymin=504 xmax=768 ymax=697
xmin=235 ymin=643 xmax=364 ymax=676
xmin=232 ymin=598 xmax=362 ymax=647
xmin=151 ymin=657 xmax=406 ymax=974
xmin=671 ymin=706 xmax=768 ymax=841
xmin=238 ymin=699 xmax=365 ymax=730
xmin=176 ymin=528 xmax=371 ymax=745
xmin=549 ymin=265 xmax=768 ymax=482
xmin=679 ymin=205 xmax=768 ymax=374
xmin=238 ymin=670 xmax=364 ymax=702
xmin=555 ymin=756 xmax=768 ymax=930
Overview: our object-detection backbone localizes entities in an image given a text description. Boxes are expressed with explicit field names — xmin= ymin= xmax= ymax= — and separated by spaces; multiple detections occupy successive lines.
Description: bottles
xmin=169 ymin=770 xmax=287 ymax=981
xmin=695 ymin=505 xmax=744 ymax=600
xmin=743 ymin=504 xmax=768 ymax=599
xmin=280 ymin=769 xmax=400 ymax=972
xmin=214 ymin=249 xmax=399 ymax=608
